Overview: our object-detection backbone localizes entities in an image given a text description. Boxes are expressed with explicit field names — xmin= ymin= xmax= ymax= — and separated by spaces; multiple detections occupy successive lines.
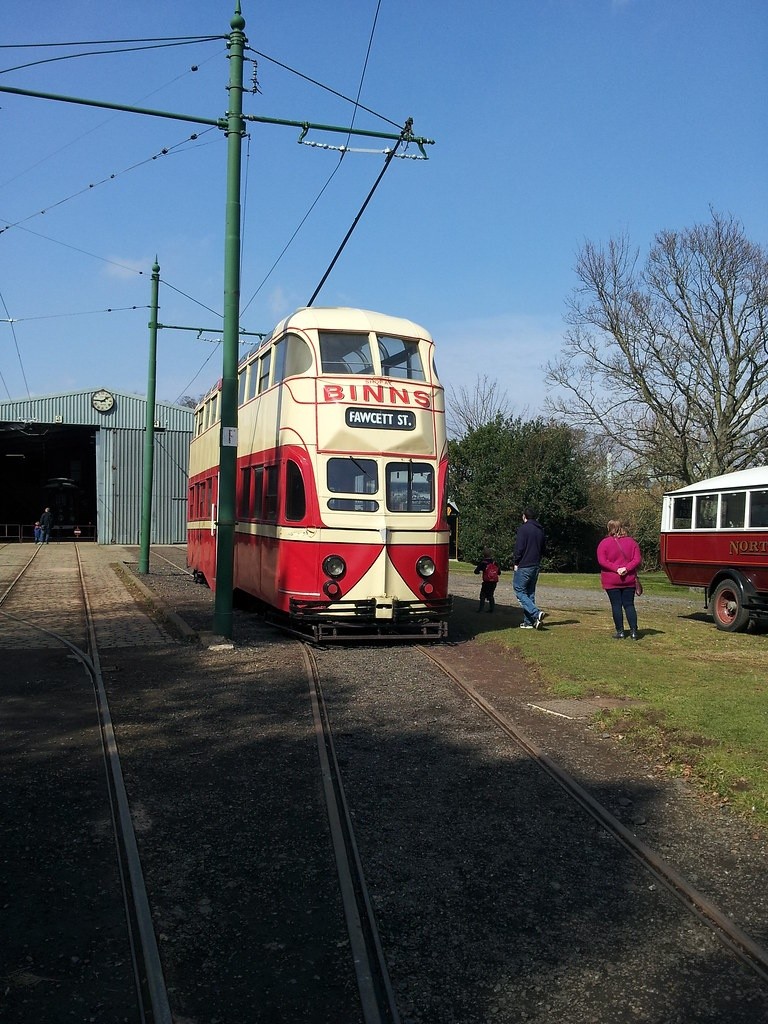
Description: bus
xmin=186 ymin=307 xmax=452 ymax=647
xmin=661 ymin=465 xmax=768 ymax=631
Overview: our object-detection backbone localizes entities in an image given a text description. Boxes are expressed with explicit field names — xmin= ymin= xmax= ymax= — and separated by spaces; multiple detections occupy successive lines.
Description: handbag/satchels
xmin=635 ymin=577 xmax=643 ymax=597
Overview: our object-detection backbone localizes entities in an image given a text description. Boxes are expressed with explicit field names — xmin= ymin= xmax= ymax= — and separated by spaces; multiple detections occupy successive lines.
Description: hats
xmin=45 ymin=508 xmax=50 ymax=513
xmin=483 ymin=547 xmax=492 ymax=559
xmin=522 ymin=508 xmax=536 ymax=519
xmin=35 ymin=522 xmax=41 ymax=526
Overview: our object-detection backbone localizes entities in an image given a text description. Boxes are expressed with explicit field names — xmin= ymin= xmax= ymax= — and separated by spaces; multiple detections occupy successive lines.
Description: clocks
xmin=91 ymin=389 xmax=114 ymax=412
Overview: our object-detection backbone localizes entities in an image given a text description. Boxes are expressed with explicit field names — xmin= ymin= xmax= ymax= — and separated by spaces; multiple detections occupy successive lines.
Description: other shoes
xmin=612 ymin=632 xmax=625 ymax=639
xmin=475 ymin=607 xmax=482 ymax=613
xmin=485 ymin=610 xmax=494 ymax=613
xmin=631 ymin=631 xmax=639 ymax=640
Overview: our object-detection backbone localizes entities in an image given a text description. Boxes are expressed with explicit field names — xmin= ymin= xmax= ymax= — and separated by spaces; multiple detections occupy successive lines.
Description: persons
xmin=513 ymin=507 xmax=546 ymax=630
xmin=34 ymin=522 xmax=41 ymax=545
xmin=597 ymin=519 xmax=642 ymax=640
xmin=37 ymin=508 xmax=53 ymax=545
xmin=474 ymin=548 xmax=501 ymax=613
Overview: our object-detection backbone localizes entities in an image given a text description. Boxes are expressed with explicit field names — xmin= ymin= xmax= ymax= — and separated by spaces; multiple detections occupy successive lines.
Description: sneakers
xmin=519 ymin=623 xmax=534 ymax=629
xmin=536 ymin=611 xmax=546 ymax=630
